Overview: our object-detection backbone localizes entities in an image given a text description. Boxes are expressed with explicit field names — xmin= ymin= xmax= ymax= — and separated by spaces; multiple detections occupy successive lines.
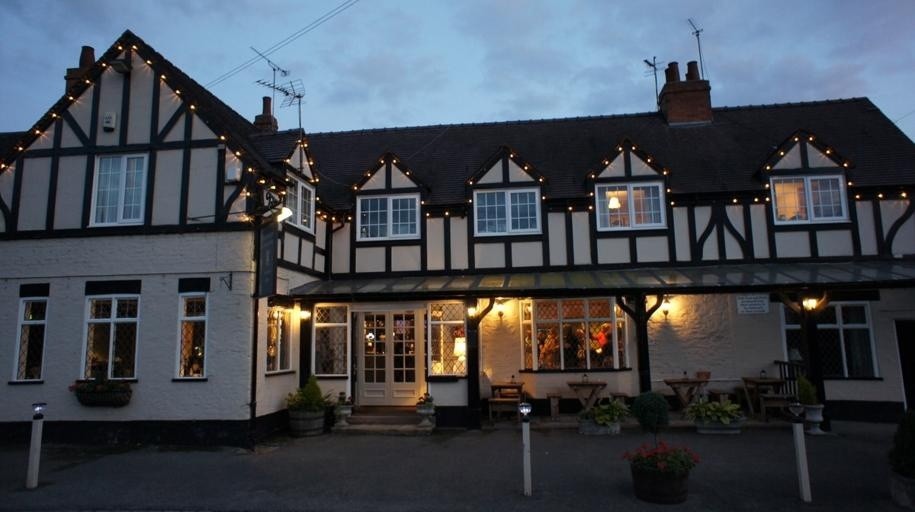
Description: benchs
xmin=611 ymin=392 xmax=628 ymax=403
xmin=734 ymin=387 xmax=797 ymax=420
xmin=546 ymin=392 xmax=563 ymax=418
xmin=489 ymin=393 xmax=521 ymax=425
xmin=708 ymin=387 xmax=728 ymax=406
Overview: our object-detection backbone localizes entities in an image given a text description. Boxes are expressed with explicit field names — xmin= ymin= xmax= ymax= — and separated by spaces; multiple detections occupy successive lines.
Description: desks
xmin=568 ymin=380 xmax=608 ymax=411
xmin=490 ymin=381 xmax=524 ymax=421
xmin=663 ymin=378 xmax=710 ymax=410
xmin=744 ymin=376 xmax=785 ymax=413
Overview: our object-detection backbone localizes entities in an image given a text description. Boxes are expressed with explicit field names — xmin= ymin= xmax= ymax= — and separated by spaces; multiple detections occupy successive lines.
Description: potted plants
xmin=796 ymin=375 xmax=829 ymax=436
xmin=682 ymin=392 xmax=749 ymax=435
xmin=574 ymin=400 xmax=632 ymax=436
xmin=286 ymin=372 xmax=335 ymax=438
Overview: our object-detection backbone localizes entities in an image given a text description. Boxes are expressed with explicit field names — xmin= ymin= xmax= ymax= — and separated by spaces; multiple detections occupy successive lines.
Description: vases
xmin=333 ymin=405 xmax=354 ymax=427
xmin=630 ymin=462 xmax=689 ymax=504
xmin=416 ymin=405 xmax=436 ymax=427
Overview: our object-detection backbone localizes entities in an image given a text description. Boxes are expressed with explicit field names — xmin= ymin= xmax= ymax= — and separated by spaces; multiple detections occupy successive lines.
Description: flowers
xmin=416 ymin=393 xmax=435 ymax=407
xmin=68 ymin=379 xmax=130 ymax=395
xmin=337 ymin=396 xmax=353 ymax=406
xmin=621 ymin=437 xmax=700 ymax=474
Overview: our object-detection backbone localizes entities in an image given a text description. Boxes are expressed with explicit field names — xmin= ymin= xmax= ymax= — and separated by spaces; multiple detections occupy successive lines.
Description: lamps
xmin=608 ymin=196 xmax=621 ymax=209
